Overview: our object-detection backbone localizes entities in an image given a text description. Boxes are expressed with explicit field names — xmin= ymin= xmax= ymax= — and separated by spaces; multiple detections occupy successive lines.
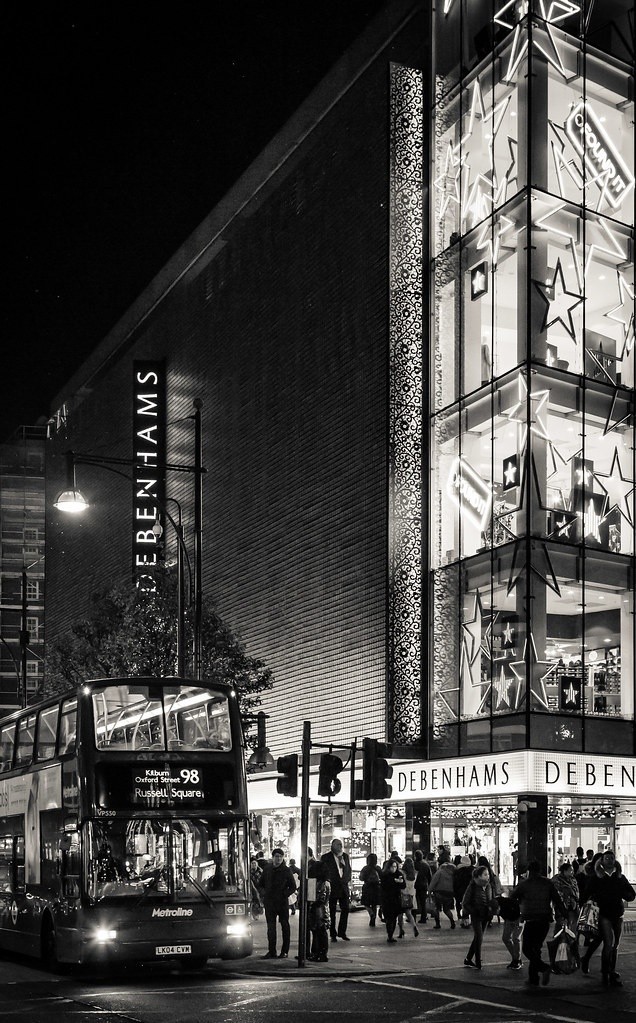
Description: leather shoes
xmin=261 ymin=951 xmax=278 ymax=958
xmin=277 ymin=952 xmax=288 ymax=958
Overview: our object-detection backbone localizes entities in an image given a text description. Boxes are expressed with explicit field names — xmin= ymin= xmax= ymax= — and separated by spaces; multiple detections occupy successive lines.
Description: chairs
xmin=0 ymin=736 xmax=217 ymax=774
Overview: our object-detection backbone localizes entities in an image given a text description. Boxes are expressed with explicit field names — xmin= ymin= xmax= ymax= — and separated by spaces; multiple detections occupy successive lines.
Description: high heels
xmin=414 ymin=926 xmax=419 ymax=937
xmin=397 ymin=930 xmax=404 ymax=938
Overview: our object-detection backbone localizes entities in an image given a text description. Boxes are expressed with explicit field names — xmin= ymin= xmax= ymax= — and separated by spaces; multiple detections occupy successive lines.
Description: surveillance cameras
xmin=517 ymin=803 xmax=527 ymax=815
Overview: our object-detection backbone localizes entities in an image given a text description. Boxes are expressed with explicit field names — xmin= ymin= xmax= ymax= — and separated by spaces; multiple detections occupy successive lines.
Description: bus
xmin=0 ymin=677 xmax=262 ymax=972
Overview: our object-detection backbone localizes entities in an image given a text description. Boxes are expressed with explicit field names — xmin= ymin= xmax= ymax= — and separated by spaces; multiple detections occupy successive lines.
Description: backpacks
xmin=573 ymin=859 xmax=592 ymax=890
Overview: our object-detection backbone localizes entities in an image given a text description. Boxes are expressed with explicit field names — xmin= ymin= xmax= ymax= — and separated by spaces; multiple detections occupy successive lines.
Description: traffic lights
xmin=275 ymin=754 xmax=298 ymax=798
xmin=318 ymin=753 xmax=342 ymax=797
xmin=361 ymin=737 xmax=393 ymax=799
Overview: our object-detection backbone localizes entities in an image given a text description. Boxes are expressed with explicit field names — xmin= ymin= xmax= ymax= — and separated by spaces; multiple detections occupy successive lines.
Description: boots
xmin=433 ymin=920 xmax=441 ymax=929
xmin=450 ymin=918 xmax=456 ymax=929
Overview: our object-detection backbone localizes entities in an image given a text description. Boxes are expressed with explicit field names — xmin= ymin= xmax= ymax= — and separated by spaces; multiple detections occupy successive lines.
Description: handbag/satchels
xmin=401 ymin=894 xmax=414 ymax=908
xmin=425 ymin=893 xmax=438 ymax=916
xmin=546 ymin=924 xmax=580 ymax=975
xmin=576 ymin=903 xmax=600 ymax=941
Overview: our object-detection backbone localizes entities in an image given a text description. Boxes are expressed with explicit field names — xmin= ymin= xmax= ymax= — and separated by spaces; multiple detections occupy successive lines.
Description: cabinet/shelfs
xmin=545 ymin=664 xmax=590 ymax=711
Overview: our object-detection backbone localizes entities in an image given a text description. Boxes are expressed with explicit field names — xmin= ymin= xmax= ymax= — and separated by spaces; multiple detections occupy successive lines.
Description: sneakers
xmin=464 ymin=959 xmax=475 ymax=967
xmin=507 ymin=960 xmax=514 ymax=969
xmin=512 ymin=959 xmax=523 ymax=969
xmin=475 ymin=965 xmax=483 ymax=970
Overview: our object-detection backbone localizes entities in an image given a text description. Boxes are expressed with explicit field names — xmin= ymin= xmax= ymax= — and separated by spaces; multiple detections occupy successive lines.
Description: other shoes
xmin=339 ymin=935 xmax=350 ymax=940
xmin=309 ymin=953 xmax=328 ymax=962
xmin=331 ymin=936 xmax=338 ymax=942
xmin=418 ymin=917 xmax=426 ymax=923
xmin=387 ymin=937 xmax=397 ymax=942
xmin=580 ymin=954 xmax=591 ymax=974
xmin=603 ymin=979 xmax=624 ymax=987
xmin=525 ymin=979 xmax=540 ymax=987
xmin=612 ymin=971 xmax=622 ymax=977
xmin=542 ymin=964 xmax=551 ymax=985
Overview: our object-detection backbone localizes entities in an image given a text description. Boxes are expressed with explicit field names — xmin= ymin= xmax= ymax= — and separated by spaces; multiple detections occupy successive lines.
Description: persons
xmin=306 ymin=847 xmax=331 ymax=961
xmin=320 ymin=839 xmax=351 ymax=942
xmin=460 ymin=866 xmax=492 ymax=969
xmin=198 ymin=730 xmax=225 ymax=751
xmin=256 ymin=848 xmax=296 ymax=959
xmin=251 ymin=851 xmax=268 ymax=921
xmin=507 ymin=847 xmax=636 ymax=986
xmin=491 ymin=897 xmax=524 ymax=970
xmin=358 ymin=845 xmax=503 ymax=942
xmin=288 ymin=859 xmax=301 ymax=915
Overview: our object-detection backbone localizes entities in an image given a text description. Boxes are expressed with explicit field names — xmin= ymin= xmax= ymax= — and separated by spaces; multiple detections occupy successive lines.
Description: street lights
xmin=53 ymin=448 xmax=205 ymax=677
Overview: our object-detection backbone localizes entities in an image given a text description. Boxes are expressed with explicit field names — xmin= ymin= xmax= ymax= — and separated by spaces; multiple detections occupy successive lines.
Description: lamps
xmin=367 ymin=809 xmax=385 ymax=829
